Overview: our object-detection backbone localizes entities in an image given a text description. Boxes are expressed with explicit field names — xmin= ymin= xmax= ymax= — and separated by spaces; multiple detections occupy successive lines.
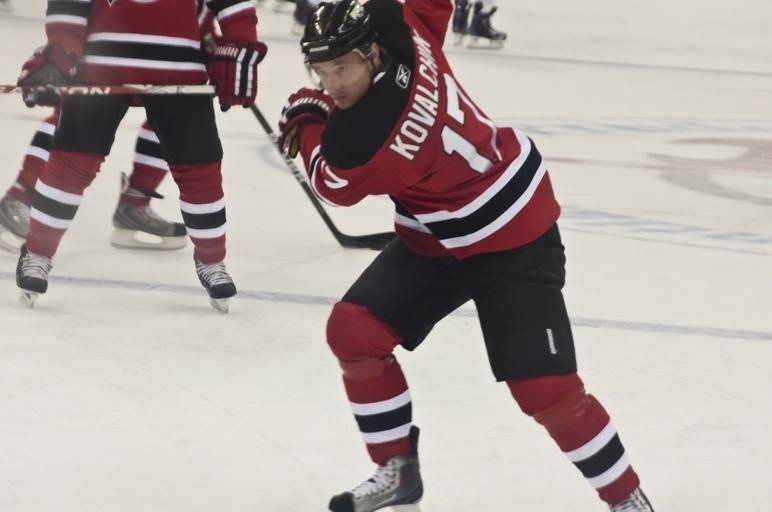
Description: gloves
xmin=276 ymin=88 xmax=334 ymax=156
xmin=208 ymin=35 xmax=267 ymax=111
xmin=17 ymin=41 xmax=83 ymax=108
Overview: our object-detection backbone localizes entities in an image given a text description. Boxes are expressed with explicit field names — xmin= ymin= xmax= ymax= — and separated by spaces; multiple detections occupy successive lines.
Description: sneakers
xmin=193 ymin=256 xmax=237 ymax=299
xmin=15 ymin=243 xmax=52 ymax=293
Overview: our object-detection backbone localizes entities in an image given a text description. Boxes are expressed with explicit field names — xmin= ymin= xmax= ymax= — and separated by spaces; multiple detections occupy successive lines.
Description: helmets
xmin=300 ymin=0 xmax=380 ymax=89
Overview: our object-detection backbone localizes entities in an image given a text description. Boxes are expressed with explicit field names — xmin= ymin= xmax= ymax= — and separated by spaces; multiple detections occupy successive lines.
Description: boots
xmin=328 ymin=426 xmax=423 ymax=512
xmin=453 ymin=0 xmax=471 ymax=34
xmin=469 ymin=1 xmax=507 ymax=41
xmin=113 ymin=171 xmax=187 ymax=237
xmin=609 ymin=489 xmax=654 ymax=512
xmin=0 ymin=194 xmax=31 ymax=238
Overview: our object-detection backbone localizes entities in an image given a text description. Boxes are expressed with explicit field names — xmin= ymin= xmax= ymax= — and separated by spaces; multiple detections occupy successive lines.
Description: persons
xmin=0 ymin=0 xmax=218 ymax=242
xmin=294 ymin=0 xmax=323 ymax=25
xmin=450 ymin=1 xmax=513 ymax=42
xmin=12 ymin=0 xmax=272 ymax=300
xmin=274 ymin=2 xmax=656 ymax=512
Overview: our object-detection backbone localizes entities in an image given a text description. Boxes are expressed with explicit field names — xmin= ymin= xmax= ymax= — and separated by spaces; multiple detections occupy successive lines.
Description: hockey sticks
xmin=251 ymin=102 xmax=398 ymax=250
